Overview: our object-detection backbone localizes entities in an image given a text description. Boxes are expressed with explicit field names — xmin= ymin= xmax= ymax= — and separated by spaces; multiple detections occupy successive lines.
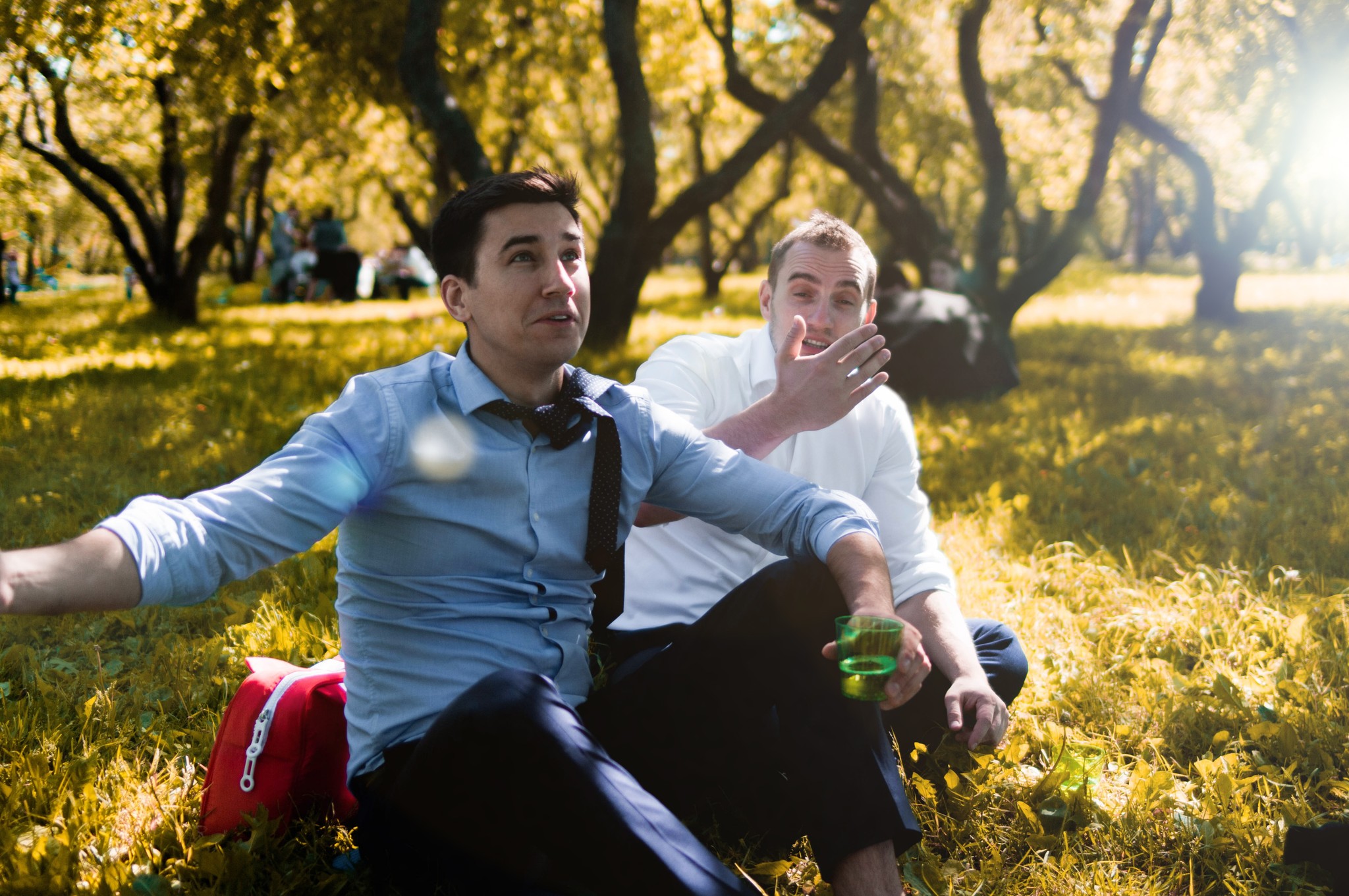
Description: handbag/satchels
xmin=199 ymin=655 xmax=356 ymax=835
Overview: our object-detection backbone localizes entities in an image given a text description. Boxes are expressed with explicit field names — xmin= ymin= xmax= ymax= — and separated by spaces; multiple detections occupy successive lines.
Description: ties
xmin=488 ymin=364 xmax=626 ymax=634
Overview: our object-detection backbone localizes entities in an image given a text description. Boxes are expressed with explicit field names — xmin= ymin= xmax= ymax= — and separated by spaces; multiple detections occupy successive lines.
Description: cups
xmin=835 ymin=616 xmax=906 ymax=701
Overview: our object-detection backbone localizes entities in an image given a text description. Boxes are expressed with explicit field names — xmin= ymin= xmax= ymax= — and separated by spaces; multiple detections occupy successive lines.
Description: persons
xmin=605 ymin=212 xmax=1028 ymax=755
xmin=0 ymin=168 xmax=933 ymax=896
xmin=264 ymin=202 xmax=438 ymax=301
xmin=8 ymin=253 xmax=20 ymax=304
